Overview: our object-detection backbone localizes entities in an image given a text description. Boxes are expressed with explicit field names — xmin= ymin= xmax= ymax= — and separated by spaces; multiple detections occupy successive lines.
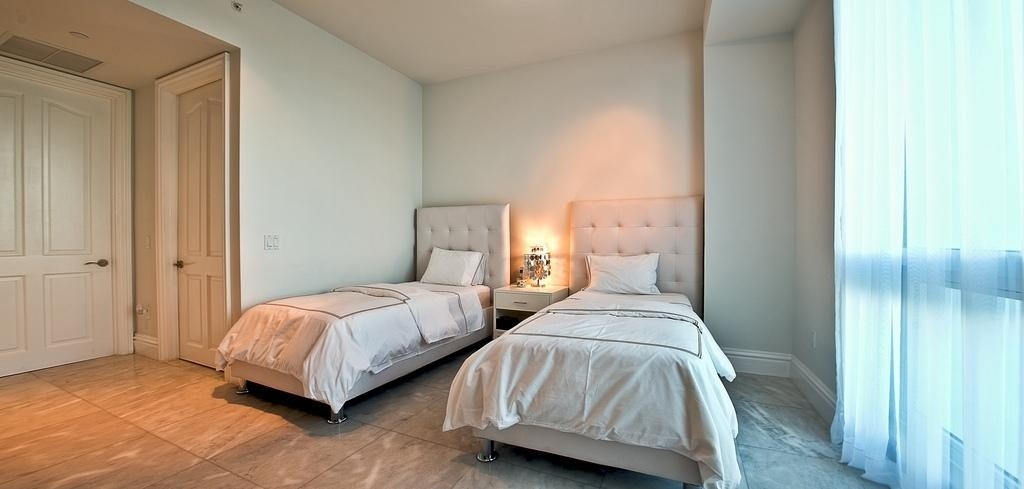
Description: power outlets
xmin=809 ymin=330 xmax=817 ymax=349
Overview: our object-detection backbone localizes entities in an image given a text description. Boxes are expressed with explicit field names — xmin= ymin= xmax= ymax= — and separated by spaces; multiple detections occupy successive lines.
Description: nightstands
xmin=492 ymin=286 xmax=567 ymax=339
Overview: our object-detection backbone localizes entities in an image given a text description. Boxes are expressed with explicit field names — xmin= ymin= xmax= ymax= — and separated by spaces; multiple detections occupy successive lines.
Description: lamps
xmin=522 ymin=232 xmax=556 ymax=288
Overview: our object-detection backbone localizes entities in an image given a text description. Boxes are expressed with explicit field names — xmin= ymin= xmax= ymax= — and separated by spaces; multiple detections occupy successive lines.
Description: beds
xmin=229 ymin=205 xmax=511 ymax=424
xmin=471 ymin=195 xmax=711 ymax=487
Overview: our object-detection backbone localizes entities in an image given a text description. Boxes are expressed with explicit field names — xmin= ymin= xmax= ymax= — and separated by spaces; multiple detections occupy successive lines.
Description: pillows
xmin=420 ymin=248 xmax=484 ymax=286
xmin=580 ymin=252 xmax=661 ymax=296
